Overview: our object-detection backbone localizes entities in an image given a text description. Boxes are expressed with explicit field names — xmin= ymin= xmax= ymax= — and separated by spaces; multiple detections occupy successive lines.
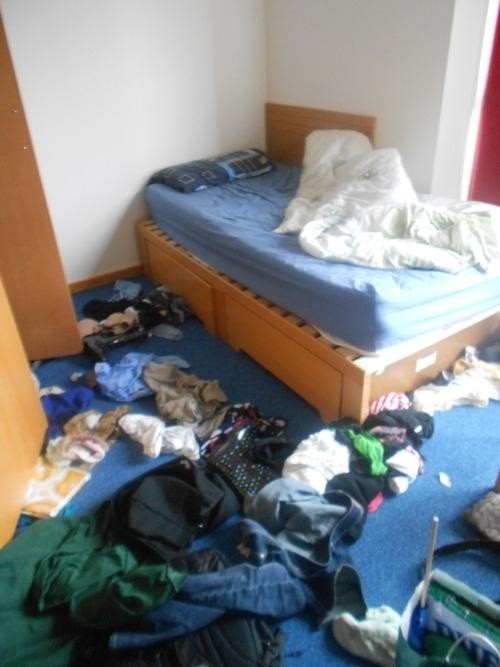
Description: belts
xmin=420 ymin=538 xmax=500 ymax=606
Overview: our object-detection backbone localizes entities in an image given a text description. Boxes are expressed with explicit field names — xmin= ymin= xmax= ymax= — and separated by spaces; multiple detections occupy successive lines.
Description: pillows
xmin=148 ymin=145 xmax=276 ymax=194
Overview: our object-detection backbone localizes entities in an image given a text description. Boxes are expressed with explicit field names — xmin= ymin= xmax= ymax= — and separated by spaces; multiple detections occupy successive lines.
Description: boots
xmin=82 ymin=327 xmax=147 ymax=364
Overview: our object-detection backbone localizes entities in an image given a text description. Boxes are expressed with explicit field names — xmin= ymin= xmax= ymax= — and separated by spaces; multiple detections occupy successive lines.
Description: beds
xmin=134 ymin=158 xmax=500 ymax=425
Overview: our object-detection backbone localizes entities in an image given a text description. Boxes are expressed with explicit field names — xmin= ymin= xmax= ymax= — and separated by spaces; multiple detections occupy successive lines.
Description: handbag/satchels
xmin=206 ymin=421 xmax=291 ymax=497
